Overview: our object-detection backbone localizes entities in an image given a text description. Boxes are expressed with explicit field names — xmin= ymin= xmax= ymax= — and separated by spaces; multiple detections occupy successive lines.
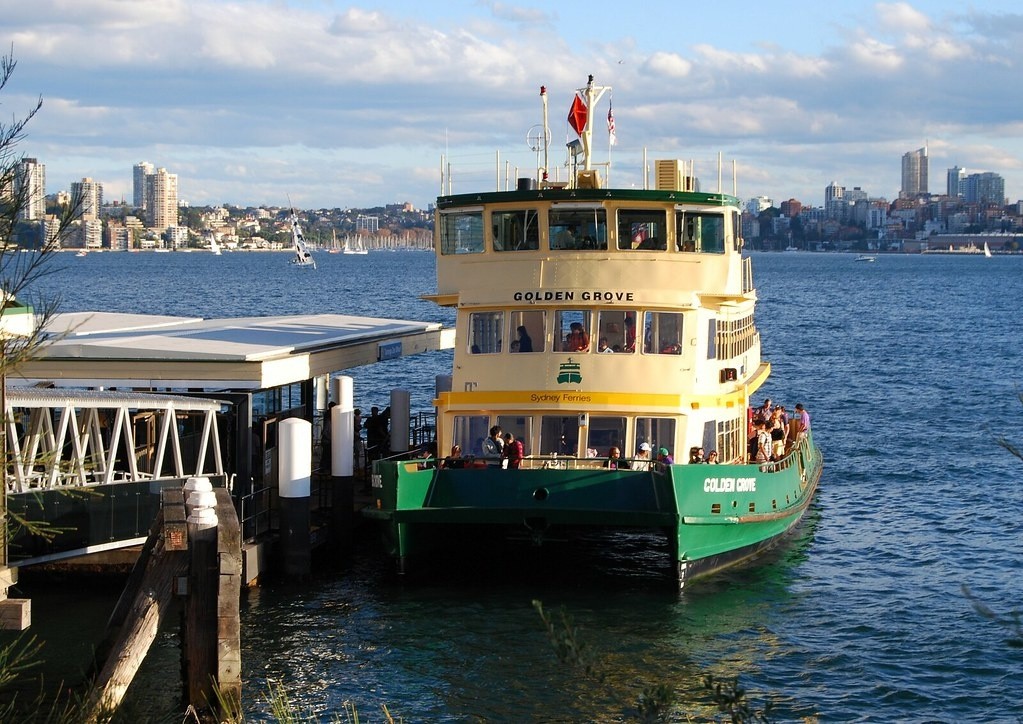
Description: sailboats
xmin=983 ymin=241 xmax=994 ymax=259
xmin=283 ymin=192 xmax=318 ymax=270
xmin=210 ymin=232 xmax=223 ymax=256
xmin=329 ymin=227 xmax=369 ymax=256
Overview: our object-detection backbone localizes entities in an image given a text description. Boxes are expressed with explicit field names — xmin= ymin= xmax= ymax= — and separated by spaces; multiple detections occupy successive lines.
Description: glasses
xmin=422 ymin=452 xmax=425 ymax=456
xmin=710 ymin=454 xmax=717 ymax=456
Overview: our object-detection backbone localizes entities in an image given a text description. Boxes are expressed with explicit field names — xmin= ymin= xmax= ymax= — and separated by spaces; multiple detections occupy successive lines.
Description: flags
xmin=606 ymin=91 xmax=617 ymax=146
xmin=568 ymin=93 xmax=589 ymax=138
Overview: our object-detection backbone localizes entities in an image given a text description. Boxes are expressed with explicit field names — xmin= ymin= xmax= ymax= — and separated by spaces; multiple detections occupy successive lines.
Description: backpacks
xmin=750 ymin=432 xmax=767 ymax=458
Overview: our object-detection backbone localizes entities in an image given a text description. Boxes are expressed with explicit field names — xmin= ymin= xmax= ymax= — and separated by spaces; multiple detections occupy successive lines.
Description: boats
xmin=354 ymin=71 xmax=825 ymax=605
xmin=153 ymin=247 xmax=170 ymax=253
xmin=95 ymin=250 xmax=103 ymax=253
xmin=74 ymin=249 xmax=91 ymax=257
xmin=854 ymin=255 xmax=878 ymax=262
xmin=183 ymin=249 xmax=192 ymax=253
xmin=785 ymin=245 xmax=799 ymax=252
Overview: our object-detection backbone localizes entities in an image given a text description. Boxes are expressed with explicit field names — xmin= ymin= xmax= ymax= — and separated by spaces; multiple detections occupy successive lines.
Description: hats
xmin=656 ymin=447 xmax=669 ymax=456
xmin=639 ymin=442 xmax=652 ymax=451
xmin=419 ymin=446 xmax=429 ymax=453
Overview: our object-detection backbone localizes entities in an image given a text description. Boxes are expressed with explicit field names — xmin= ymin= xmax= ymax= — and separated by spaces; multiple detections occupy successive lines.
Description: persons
xmin=462 ymin=454 xmax=488 ymax=469
xmin=654 ymin=447 xmax=675 ymax=473
xmin=500 ymin=432 xmax=523 ymax=468
xmin=471 ymin=345 xmax=481 ymax=353
xmin=581 ymin=235 xmax=598 ymax=249
xmin=363 ymin=407 xmax=391 ymax=475
xmin=410 ymin=446 xmax=437 ymax=469
xmin=498 ymin=325 xmax=533 ymax=352
xmin=550 ymin=223 xmax=577 ymax=249
xmin=602 ymin=447 xmax=630 ymax=469
xmin=749 ymin=398 xmax=809 ymax=472
xmin=316 ymin=401 xmax=337 ymax=472
xmin=482 ymin=425 xmax=504 ymax=468
xmin=600 ymin=242 xmax=607 ymax=250
xmin=441 ymin=443 xmax=464 ymax=469
xmin=645 ymin=322 xmax=681 ymax=353
xmin=630 ymin=442 xmax=652 ymax=471
xmin=354 ymin=408 xmax=363 ymax=471
xmin=688 ymin=447 xmax=719 ymax=464
xmin=566 ymin=316 xmax=636 ymax=353
